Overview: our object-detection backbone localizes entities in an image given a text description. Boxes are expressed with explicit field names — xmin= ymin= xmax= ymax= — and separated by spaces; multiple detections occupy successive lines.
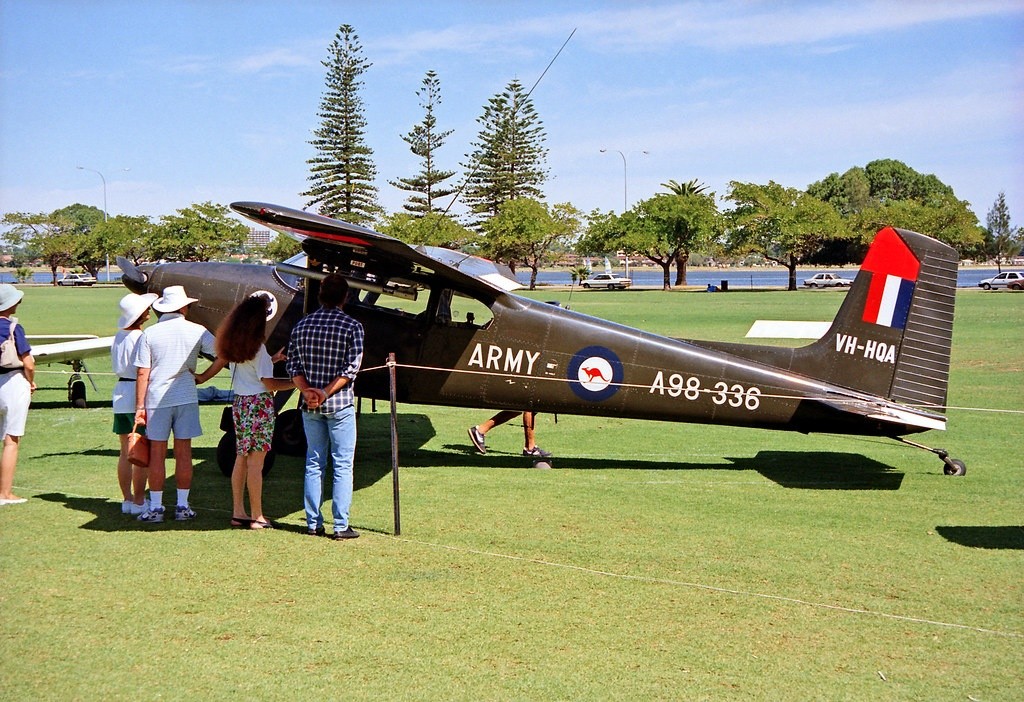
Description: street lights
xmin=600 ymin=150 xmax=629 ymax=278
xmin=77 ymin=165 xmax=110 ymax=283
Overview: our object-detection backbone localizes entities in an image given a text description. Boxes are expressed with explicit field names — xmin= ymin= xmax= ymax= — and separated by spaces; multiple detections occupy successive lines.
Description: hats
xmin=0 ymin=285 xmax=24 ymax=311
xmin=117 ymin=293 xmax=158 ymax=330
xmin=153 ymin=286 xmax=198 ymax=313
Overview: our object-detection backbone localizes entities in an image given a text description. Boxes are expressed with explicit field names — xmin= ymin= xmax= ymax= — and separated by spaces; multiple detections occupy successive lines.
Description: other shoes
xmin=333 ymin=527 xmax=359 ymax=538
xmin=308 ymin=526 xmax=325 ymax=535
xmin=0 ymin=498 xmax=28 ymax=505
xmin=231 ymin=518 xmax=273 ymax=529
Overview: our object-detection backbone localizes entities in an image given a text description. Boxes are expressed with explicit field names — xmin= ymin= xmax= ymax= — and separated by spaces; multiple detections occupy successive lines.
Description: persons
xmin=215 ymin=296 xmax=296 ymax=530
xmin=134 ymin=286 xmax=228 ymax=523
xmin=111 ymin=293 xmax=166 ymax=515
xmin=286 ymin=274 xmax=364 ymax=539
xmin=0 ymin=284 xmax=36 ymax=505
xmin=468 ymin=411 xmax=551 ymax=456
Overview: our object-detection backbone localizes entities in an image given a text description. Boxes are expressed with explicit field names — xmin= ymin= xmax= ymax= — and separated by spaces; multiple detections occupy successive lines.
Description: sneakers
xmin=137 ymin=507 xmax=163 ymax=522
xmin=174 ymin=505 xmax=198 ymax=520
xmin=122 ymin=501 xmax=131 ymax=513
xmin=523 ymin=446 xmax=551 ymax=456
xmin=131 ymin=503 xmax=165 ymax=514
xmin=468 ymin=427 xmax=486 ymax=454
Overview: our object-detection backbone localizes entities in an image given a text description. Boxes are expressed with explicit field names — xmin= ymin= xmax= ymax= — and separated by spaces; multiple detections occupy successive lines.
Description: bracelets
xmin=321 ymin=388 xmax=329 ymax=399
xmin=136 ymin=407 xmax=145 ymax=410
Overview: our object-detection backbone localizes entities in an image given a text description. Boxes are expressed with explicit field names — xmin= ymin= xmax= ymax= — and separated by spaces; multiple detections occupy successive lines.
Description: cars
xmin=1007 ymin=279 xmax=1024 ymax=291
xmin=57 ymin=273 xmax=97 ymax=286
xmin=581 ymin=273 xmax=633 ymax=290
xmin=803 ymin=272 xmax=853 ymax=287
xmin=978 ymin=271 xmax=1024 ymax=290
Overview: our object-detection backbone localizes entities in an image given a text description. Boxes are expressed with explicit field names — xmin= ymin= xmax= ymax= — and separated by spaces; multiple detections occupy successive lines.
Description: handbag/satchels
xmin=220 ymin=406 xmax=235 ymax=432
xmin=0 ymin=318 xmax=35 ymax=368
xmin=127 ymin=423 xmax=148 ymax=468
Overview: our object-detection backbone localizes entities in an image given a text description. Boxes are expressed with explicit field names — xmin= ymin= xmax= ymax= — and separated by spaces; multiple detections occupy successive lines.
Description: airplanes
xmin=113 ymin=201 xmax=966 ymax=478
xmin=24 ymin=335 xmax=117 ymax=408
